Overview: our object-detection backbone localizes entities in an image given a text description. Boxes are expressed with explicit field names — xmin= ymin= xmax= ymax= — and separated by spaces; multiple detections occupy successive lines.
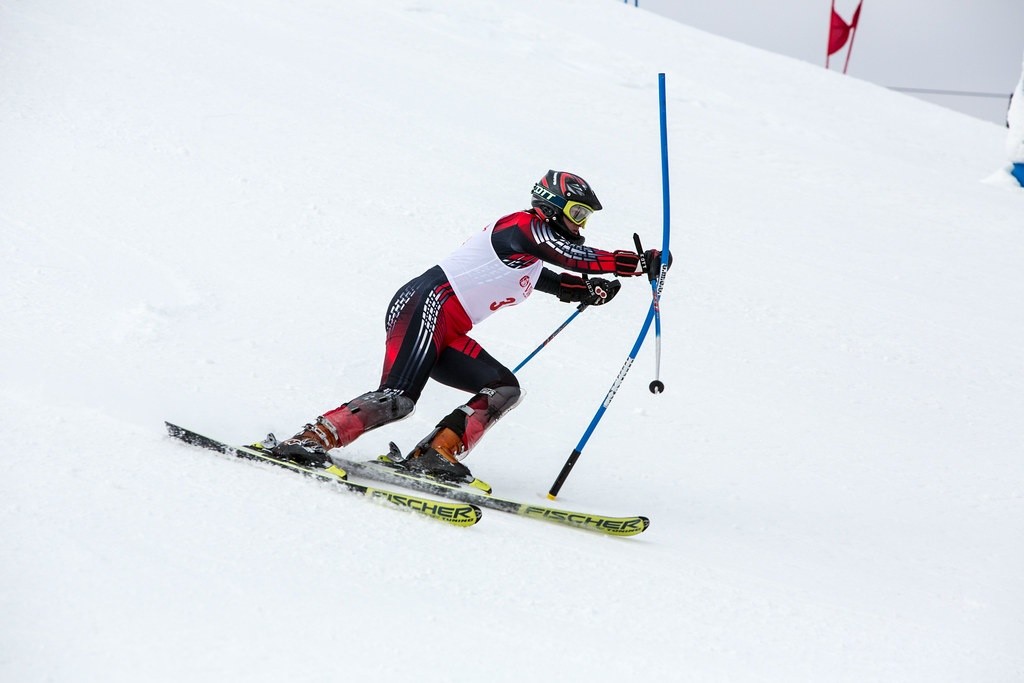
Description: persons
xmin=272 ymin=169 xmax=673 ymax=493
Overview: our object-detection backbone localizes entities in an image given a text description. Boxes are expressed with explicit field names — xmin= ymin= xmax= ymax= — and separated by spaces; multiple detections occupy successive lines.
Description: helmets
xmin=531 ymin=170 xmax=602 ymax=245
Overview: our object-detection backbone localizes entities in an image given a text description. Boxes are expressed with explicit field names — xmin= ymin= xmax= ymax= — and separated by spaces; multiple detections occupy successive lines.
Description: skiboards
xmin=163 ymin=418 xmax=650 ymax=537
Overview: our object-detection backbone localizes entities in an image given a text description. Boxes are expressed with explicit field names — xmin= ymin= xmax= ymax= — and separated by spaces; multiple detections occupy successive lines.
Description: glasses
xmin=532 ymin=184 xmax=593 ymax=229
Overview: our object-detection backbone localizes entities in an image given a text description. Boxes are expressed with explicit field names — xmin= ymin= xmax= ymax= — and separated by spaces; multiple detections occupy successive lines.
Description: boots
xmin=275 ymin=421 xmax=349 ymax=481
xmin=407 ymin=428 xmax=492 ymax=493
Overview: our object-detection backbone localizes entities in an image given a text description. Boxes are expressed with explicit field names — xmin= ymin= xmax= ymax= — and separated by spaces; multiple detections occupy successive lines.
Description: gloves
xmin=643 ymin=249 xmax=673 ymax=281
xmin=584 ymin=277 xmax=615 ymax=306
